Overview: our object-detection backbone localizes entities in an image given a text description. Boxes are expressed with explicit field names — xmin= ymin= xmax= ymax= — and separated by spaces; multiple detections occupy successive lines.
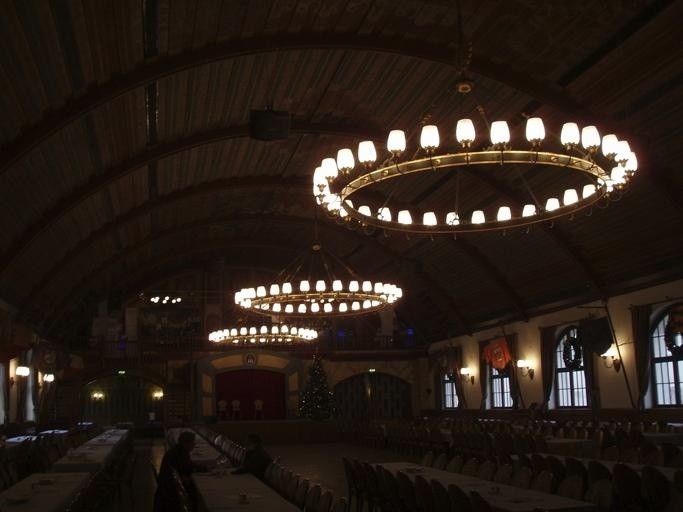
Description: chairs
xmin=0 ymin=399 xmax=683 ymax=512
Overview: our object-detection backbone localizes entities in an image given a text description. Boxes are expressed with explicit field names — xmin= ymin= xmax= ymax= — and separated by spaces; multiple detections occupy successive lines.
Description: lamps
xmin=517 ymin=360 xmax=534 ymax=379
xmin=233 ymin=209 xmax=404 ymax=317
xmin=208 ymin=320 xmax=318 ymax=343
xmin=10 ymin=366 xmax=29 ymax=385
xmin=601 ymin=344 xmax=620 ymax=373
xmin=461 ymin=367 xmax=474 ymax=384
xmin=310 ymin=0 xmax=639 ymax=239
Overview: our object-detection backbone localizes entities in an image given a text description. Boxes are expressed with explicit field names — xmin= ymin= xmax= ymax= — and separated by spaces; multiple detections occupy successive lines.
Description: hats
xmin=179 ymin=431 xmax=196 ymax=440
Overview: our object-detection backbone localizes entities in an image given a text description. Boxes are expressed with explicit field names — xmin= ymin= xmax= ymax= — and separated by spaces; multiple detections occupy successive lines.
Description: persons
xmin=150 ymin=431 xmax=215 ymax=512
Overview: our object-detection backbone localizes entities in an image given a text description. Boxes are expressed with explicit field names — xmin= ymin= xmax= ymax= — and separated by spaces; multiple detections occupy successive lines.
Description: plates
xmin=6 ymin=495 xmax=32 ymax=503
xmin=36 ymin=478 xmax=56 ymax=485
